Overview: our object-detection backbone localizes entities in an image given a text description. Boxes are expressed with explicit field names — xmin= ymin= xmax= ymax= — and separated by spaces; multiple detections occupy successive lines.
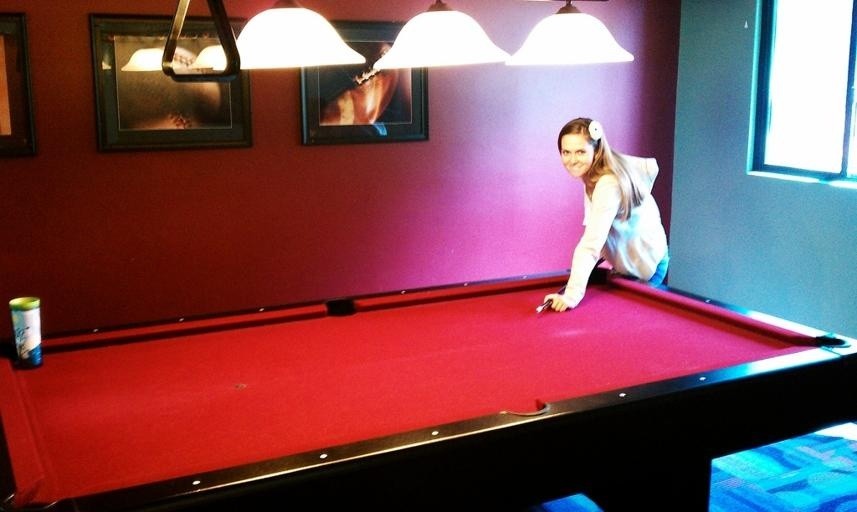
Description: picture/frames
xmin=297 ymin=21 xmax=428 ymax=147
xmin=0 ymin=11 xmax=37 ymax=160
xmin=87 ymin=12 xmax=253 ymax=154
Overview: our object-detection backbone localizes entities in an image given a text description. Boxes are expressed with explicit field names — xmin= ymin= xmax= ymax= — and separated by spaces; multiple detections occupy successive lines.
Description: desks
xmin=0 ymin=266 xmax=857 ymax=512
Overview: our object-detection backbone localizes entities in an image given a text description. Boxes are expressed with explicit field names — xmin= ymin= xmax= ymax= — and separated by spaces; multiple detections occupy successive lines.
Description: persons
xmin=543 ymin=118 xmax=669 ymax=312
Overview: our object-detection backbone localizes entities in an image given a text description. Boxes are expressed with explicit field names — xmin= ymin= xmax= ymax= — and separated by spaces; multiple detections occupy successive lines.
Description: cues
xmin=536 ymin=256 xmax=607 ymax=314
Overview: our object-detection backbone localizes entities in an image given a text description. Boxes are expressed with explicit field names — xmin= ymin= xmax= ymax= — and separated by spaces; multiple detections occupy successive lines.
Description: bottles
xmin=8 ymin=296 xmax=42 ymax=367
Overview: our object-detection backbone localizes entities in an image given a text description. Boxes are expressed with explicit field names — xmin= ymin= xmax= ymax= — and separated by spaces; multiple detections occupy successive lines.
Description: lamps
xmin=504 ymin=2 xmax=634 ymax=67
xmin=211 ymin=1 xmax=366 ymax=70
xmin=372 ymin=0 xmax=510 ymax=71
xmin=120 ymin=41 xmax=164 ymax=72
